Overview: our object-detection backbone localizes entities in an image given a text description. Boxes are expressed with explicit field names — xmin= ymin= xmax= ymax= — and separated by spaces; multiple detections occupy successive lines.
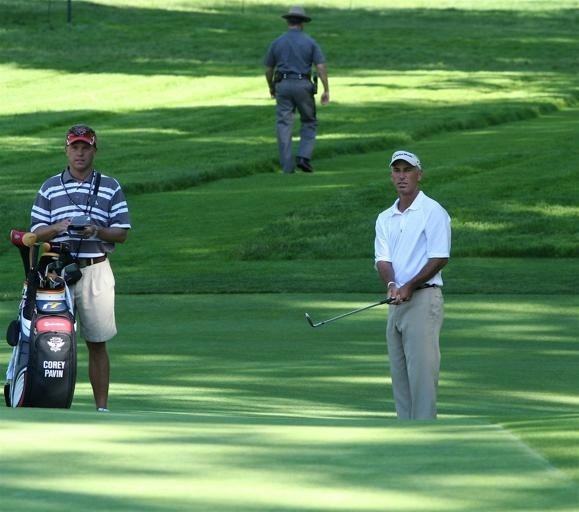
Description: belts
xmin=413 ymin=282 xmax=435 ymax=289
xmin=81 ymin=254 xmax=107 ymax=267
xmin=283 ymin=72 xmax=310 ymax=80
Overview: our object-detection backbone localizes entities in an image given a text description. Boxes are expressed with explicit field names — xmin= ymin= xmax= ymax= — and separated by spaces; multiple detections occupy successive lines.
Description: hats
xmin=282 ymin=7 xmax=312 ymax=24
xmin=64 ymin=126 xmax=98 ymax=146
xmin=390 ymin=151 xmax=423 ymax=169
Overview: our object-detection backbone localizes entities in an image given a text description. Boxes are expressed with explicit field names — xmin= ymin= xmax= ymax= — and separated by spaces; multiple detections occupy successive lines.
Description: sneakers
xmin=296 ymin=157 xmax=312 ymax=171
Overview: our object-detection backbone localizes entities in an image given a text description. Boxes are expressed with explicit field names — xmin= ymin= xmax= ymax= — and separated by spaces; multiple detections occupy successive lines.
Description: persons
xmin=374 ymin=151 xmax=451 ymax=420
xmin=262 ymin=6 xmax=329 ymax=173
xmin=31 ymin=125 xmax=131 ymax=413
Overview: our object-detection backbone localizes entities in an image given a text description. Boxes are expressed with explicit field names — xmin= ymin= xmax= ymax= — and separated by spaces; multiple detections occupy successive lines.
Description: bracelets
xmin=91 ymin=226 xmax=99 ymax=239
xmin=388 ymin=281 xmax=395 ymax=288
